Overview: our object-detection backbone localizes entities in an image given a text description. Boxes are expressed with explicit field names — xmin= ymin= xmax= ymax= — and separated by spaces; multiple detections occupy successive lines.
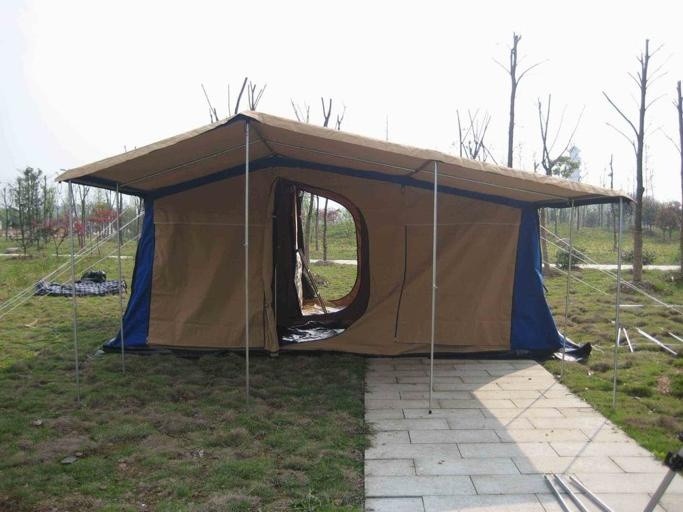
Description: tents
xmin=56 ymin=112 xmax=639 ymax=357
